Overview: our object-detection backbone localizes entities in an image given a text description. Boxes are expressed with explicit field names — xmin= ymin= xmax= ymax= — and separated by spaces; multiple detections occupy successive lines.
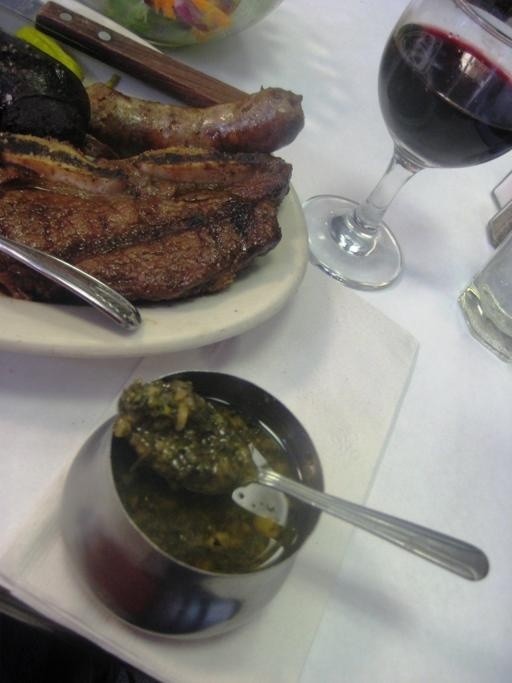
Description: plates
xmin=0 ymin=2 xmax=309 ymax=361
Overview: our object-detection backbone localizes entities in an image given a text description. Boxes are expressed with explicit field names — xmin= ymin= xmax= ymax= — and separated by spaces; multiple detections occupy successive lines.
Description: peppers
xmin=15 ymin=26 xmax=121 ymax=90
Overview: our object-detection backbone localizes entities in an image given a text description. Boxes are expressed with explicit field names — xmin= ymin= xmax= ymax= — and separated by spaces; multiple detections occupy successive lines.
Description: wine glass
xmin=304 ymin=1 xmax=512 ymax=291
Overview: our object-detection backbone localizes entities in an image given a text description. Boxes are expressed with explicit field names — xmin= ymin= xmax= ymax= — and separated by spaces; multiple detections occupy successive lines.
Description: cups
xmin=459 ymin=228 xmax=512 ymax=364
xmin=60 ymin=370 xmax=325 ymax=640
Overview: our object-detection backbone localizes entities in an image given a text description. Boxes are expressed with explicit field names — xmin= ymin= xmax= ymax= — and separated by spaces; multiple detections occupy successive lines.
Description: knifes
xmin=0 ymin=0 xmax=251 ymax=107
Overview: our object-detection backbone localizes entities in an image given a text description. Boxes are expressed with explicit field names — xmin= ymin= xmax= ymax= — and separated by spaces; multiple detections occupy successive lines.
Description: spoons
xmin=0 ymin=231 xmax=145 ymax=336
xmin=117 ymin=383 xmax=489 ymax=582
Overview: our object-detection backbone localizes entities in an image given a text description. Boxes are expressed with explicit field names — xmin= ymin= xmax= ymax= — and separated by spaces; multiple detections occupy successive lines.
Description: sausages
xmin=86 ymin=81 xmax=305 ymax=154
